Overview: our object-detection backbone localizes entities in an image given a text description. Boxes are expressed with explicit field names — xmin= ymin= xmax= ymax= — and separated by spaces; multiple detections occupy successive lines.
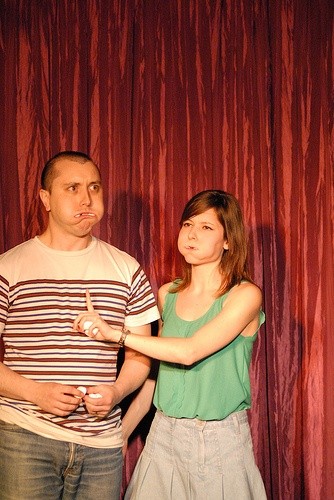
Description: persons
xmin=0 ymin=149 xmax=160 ymax=500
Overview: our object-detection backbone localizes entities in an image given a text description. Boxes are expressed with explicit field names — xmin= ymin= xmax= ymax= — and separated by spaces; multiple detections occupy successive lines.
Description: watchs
xmin=118 ymin=328 xmax=131 ymax=346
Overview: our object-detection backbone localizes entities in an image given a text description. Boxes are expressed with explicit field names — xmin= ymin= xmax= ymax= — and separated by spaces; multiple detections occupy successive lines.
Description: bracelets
xmin=73 ymin=189 xmax=267 ymax=500
